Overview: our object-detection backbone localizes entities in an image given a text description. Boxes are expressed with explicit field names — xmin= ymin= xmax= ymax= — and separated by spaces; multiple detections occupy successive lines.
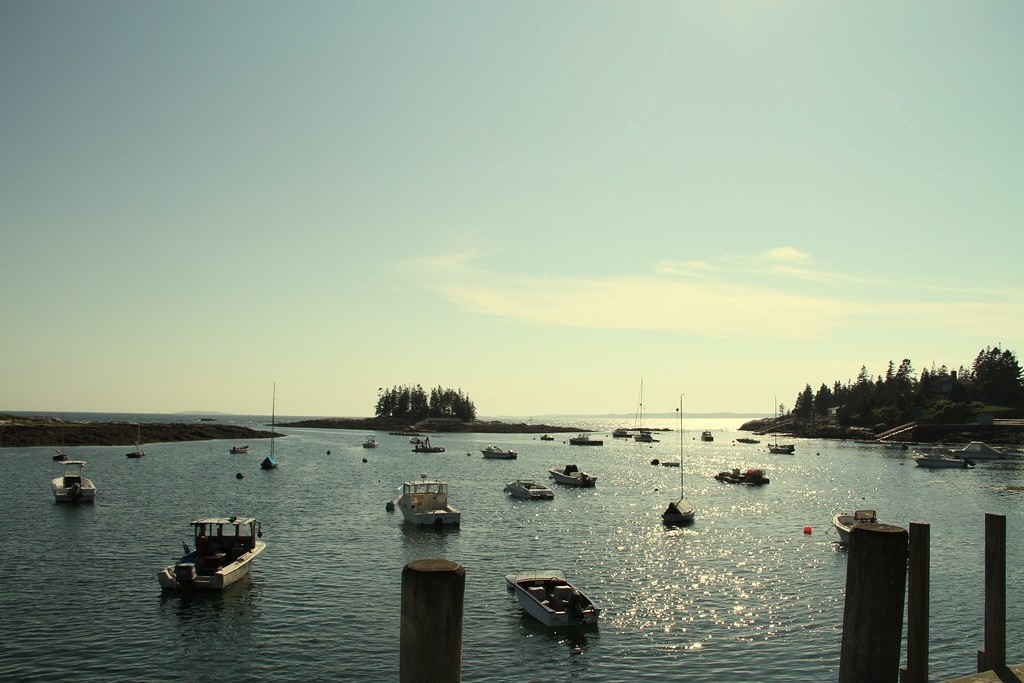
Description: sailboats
xmin=634 ymin=377 xmax=660 ymax=442
xmin=260 ymin=381 xmax=279 ymax=468
xmin=664 ymin=393 xmax=697 ymax=520
xmin=53 ymin=411 xmax=69 ymax=461
xmin=125 ymin=416 xmax=145 ymax=457
xmin=769 ymin=393 xmax=795 ymax=453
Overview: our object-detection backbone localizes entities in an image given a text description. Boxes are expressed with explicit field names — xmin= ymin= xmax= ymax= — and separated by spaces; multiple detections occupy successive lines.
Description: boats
xmin=229 ymin=443 xmax=250 ymax=454
xmin=409 ymin=436 xmax=423 ymax=444
xmin=736 ymin=436 xmax=758 ymax=444
xmin=396 ymin=473 xmax=461 ymax=528
xmin=548 ymin=465 xmax=596 ymax=486
xmin=568 ymin=433 xmax=603 ymax=446
xmin=363 ymin=434 xmax=378 ymax=448
xmin=753 ymin=431 xmax=765 ymax=435
xmin=52 ymin=461 xmax=96 ymax=502
xmin=829 ymin=507 xmax=911 ymax=546
xmin=612 ymin=429 xmax=632 ymax=437
xmin=505 ymin=569 xmax=601 ymax=630
xmin=701 ymin=430 xmax=711 ymax=441
xmin=412 ymin=437 xmax=445 ymax=453
xmin=715 ymin=468 xmax=769 ymax=486
xmin=884 ymin=441 xmax=1009 ymax=468
xmin=541 ymin=434 xmax=554 ymax=440
xmin=481 ymin=445 xmax=517 ymax=459
xmin=505 ymin=479 xmax=555 ymax=498
xmin=156 ymin=514 xmax=266 ymax=593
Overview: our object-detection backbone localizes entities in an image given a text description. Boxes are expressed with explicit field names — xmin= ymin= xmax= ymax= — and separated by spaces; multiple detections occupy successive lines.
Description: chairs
xmin=548 ymin=586 xmax=572 ymax=604
xmin=528 ymin=586 xmax=550 ymax=605
